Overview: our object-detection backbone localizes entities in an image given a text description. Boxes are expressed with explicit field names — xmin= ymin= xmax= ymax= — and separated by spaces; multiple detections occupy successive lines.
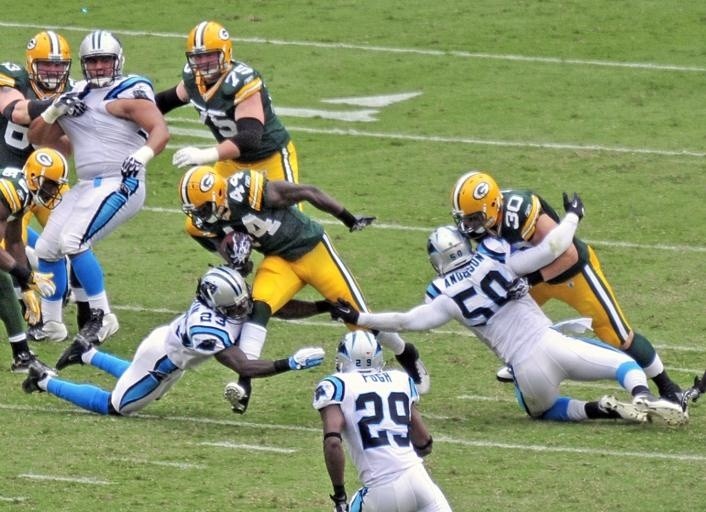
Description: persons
xmin=156 ymin=18 xmax=303 ymax=251
xmin=181 ymin=165 xmax=430 ymax=415
xmin=0 ymin=31 xmax=171 ymax=374
xmin=314 ymin=328 xmax=452 ymax=512
xmin=454 ymin=170 xmax=687 ymax=412
xmin=325 ymin=190 xmax=691 ymax=425
xmin=23 ymin=265 xmax=332 ymax=416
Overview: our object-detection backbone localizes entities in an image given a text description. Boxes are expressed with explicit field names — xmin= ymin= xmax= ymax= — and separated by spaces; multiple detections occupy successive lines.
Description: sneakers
xmin=24 ymin=318 xmax=69 ymax=344
xmin=658 ymin=381 xmax=689 ymax=414
xmin=79 ymin=310 xmax=120 ymax=346
xmin=11 ymin=356 xmax=59 ymax=378
xmin=633 ymin=387 xmax=690 ymax=427
xmin=56 ymin=331 xmax=93 ymax=371
xmin=21 ymin=363 xmax=55 ymax=394
xmin=398 ymin=344 xmax=430 ymax=396
xmin=496 ymin=364 xmax=513 ymax=381
xmin=598 ymin=392 xmax=648 ymax=423
xmin=223 ymin=382 xmax=251 ymax=415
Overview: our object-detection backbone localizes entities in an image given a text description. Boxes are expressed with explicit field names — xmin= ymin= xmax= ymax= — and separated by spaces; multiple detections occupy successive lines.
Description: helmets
xmin=335 ymin=331 xmax=385 ymax=373
xmin=426 ymin=226 xmax=474 ymax=276
xmin=78 ymin=31 xmax=123 ymax=87
xmin=452 ymin=171 xmax=503 ymax=238
xmin=24 ymin=32 xmax=71 ymax=94
xmin=178 ymin=166 xmax=228 ymax=224
xmin=22 ymin=148 xmax=68 ymax=209
xmin=198 ymin=266 xmax=251 ymax=321
xmin=182 ymin=21 xmax=232 ymax=86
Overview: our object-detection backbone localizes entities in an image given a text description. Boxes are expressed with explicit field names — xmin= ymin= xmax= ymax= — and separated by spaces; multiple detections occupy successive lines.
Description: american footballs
xmin=226 ymin=231 xmax=252 ymax=258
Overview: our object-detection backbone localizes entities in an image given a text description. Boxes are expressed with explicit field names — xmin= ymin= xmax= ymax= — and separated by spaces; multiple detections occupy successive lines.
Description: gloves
xmin=121 ymin=147 xmax=154 ymax=178
xmin=328 ymin=297 xmax=359 ymax=327
xmin=329 ymin=491 xmax=349 ymax=512
xmin=172 ymin=146 xmax=219 ymax=170
xmin=290 ymin=344 xmax=325 ymax=371
xmin=350 ymin=213 xmax=376 ymax=234
xmin=20 ymin=292 xmax=41 ymax=326
xmin=41 ymin=96 xmax=87 ymax=126
xmin=26 ymin=270 xmax=56 ymax=300
xmin=562 ymin=191 xmax=584 ymax=221
xmin=505 ymin=276 xmax=532 ymax=303
